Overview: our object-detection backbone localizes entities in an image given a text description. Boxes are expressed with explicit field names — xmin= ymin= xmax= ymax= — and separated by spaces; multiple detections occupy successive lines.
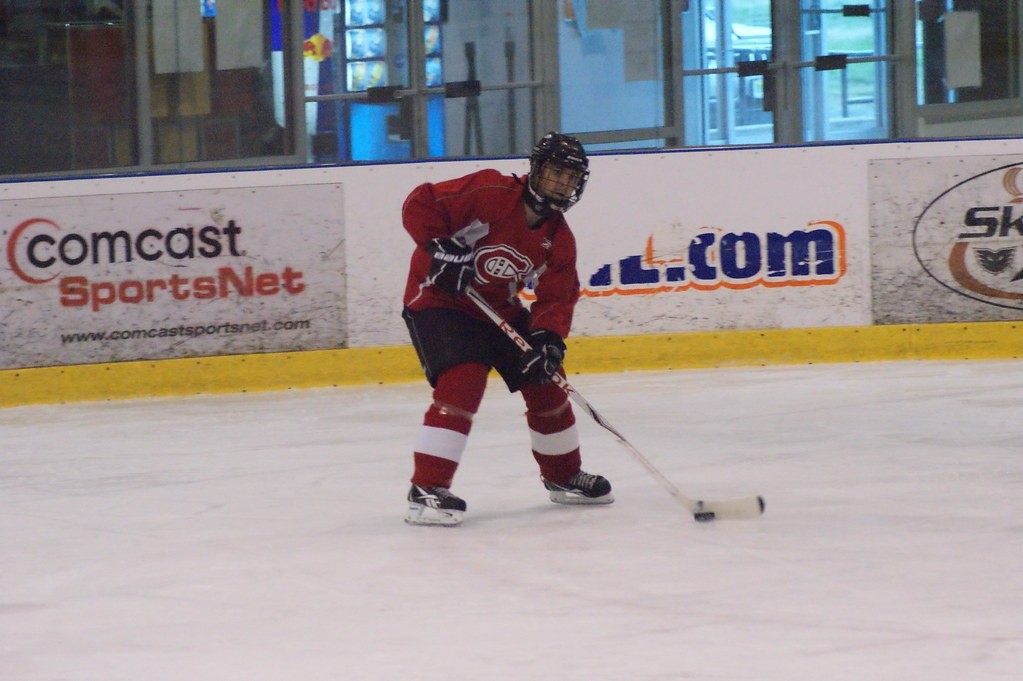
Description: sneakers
xmin=543 ymin=467 xmax=613 ymax=504
xmin=405 ymin=482 xmax=466 ymax=525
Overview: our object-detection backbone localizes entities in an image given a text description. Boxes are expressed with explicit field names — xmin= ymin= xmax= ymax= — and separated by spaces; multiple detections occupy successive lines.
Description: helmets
xmin=525 ymin=130 xmax=590 ymax=218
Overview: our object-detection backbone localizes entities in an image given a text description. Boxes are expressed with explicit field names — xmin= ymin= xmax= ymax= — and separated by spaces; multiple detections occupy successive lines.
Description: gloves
xmin=517 ymin=329 xmax=566 ymax=386
xmin=424 ymin=236 xmax=475 ymax=296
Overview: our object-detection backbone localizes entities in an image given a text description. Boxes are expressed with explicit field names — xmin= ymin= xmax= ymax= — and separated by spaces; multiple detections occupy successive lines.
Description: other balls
xmin=694 ymin=513 xmax=714 ymax=521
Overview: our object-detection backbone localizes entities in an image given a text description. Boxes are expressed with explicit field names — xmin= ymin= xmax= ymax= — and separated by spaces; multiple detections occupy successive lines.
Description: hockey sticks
xmin=464 ymin=283 xmax=765 ymax=518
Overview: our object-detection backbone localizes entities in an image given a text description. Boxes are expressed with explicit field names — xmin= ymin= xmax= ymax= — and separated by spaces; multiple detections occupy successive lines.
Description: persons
xmin=401 ymin=130 xmax=615 ymax=526
